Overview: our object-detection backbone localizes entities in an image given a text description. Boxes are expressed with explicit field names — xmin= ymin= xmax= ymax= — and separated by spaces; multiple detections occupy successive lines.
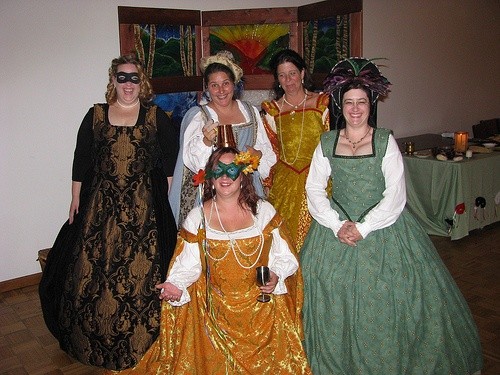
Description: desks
xmin=401 ymin=142 xmax=500 ymax=240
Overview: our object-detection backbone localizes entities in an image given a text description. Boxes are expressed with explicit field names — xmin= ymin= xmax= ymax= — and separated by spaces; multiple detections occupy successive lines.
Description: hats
xmin=200 ymin=49 xmax=245 ymax=80
xmin=322 ymin=53 xmax=394 ymax=111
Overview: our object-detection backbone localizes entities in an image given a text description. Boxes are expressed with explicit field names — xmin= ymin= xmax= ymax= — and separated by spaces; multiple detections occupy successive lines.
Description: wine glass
xmin=256 ymin=266 xmax=271 ymax=302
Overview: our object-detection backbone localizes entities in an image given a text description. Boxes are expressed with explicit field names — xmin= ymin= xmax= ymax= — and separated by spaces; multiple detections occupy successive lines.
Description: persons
xmin=36 ymin=54 xmax=180 ymax=372
xmin=295 ymin=58 xmax=484 ymax=374
xmin=257 ymin=48 xmax=331 ymax=257
xmin=168 ymin=54 xmax=277 ymax=234
xmin=113 ymin=145 xmax=313 ymax=374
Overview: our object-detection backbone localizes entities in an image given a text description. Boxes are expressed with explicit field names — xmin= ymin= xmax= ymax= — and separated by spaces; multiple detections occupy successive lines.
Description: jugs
xmin=213 ymin=124 xmax=236 ymax=148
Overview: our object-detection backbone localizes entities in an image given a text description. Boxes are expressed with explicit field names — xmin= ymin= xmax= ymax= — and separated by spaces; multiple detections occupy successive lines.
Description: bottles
xmin=408 ymin=141 xmax=412 ymax=155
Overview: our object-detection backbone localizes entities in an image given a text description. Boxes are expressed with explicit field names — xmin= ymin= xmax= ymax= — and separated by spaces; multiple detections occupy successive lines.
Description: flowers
xmin=233 ymin=151 xmax=260 ymax=175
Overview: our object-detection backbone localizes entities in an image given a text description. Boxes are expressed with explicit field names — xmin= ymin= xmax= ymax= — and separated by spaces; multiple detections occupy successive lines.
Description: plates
xmin=413 ymin=150 xmax=432 ymax=158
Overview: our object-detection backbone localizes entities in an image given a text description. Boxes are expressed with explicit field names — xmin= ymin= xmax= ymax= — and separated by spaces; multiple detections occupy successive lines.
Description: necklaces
xmin=277 ymin=88 xmax=308 ymax=164
xmin=116 ymin=98 xmax=140 ymax=109
xmin=344 ymin=126 xmax=371 ymax=149
xmin=203 ymin=194 xmax=264 ymax=268
xmin=282 ymin=87 xmax=307 ymax=110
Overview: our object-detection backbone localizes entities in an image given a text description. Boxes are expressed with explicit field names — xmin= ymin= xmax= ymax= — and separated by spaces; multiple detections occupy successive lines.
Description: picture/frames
xmin=297 ymin=0 xmax=364 ymax=92
xmin=118 ymin=6 xmax=203 ymax=94
xmin=202 ymin=7 xmax=299 ymax=90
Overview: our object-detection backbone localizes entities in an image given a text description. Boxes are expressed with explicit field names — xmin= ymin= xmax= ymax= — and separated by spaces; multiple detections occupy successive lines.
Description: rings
xmin=203 ymin=127 xmax=206 ymax=130
xmin=206 ymin=129 xmax=210 ymax=133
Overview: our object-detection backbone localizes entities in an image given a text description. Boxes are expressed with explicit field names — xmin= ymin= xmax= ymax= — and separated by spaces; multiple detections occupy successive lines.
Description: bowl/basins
xmin=482 ymin=144 xmax=497 ymax=148
